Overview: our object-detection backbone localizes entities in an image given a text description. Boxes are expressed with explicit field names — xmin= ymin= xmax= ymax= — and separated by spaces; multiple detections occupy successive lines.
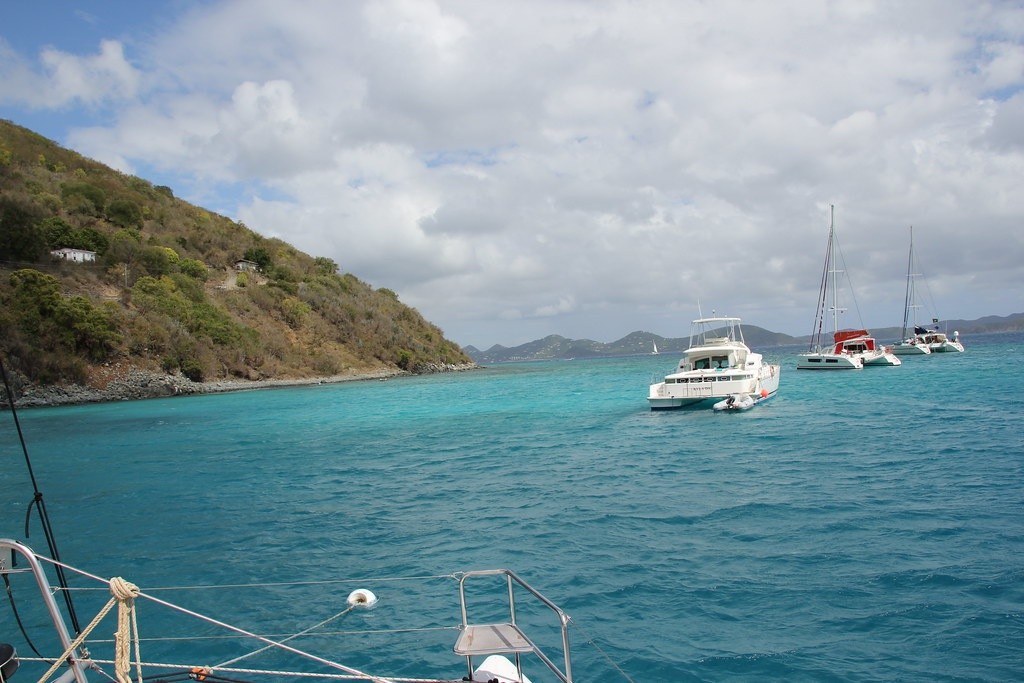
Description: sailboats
xmin=648 ymin=339 xmax=660 ymax=357
xmin=794 ymin=203 xmax=964 ymax=371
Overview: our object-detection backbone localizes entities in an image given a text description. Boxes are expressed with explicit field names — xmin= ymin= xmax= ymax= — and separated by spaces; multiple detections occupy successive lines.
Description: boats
xmin=646 ymin=300 xmax=781 ymax=411
xmin=712 ymin=393 xmax=754 ymax=411
